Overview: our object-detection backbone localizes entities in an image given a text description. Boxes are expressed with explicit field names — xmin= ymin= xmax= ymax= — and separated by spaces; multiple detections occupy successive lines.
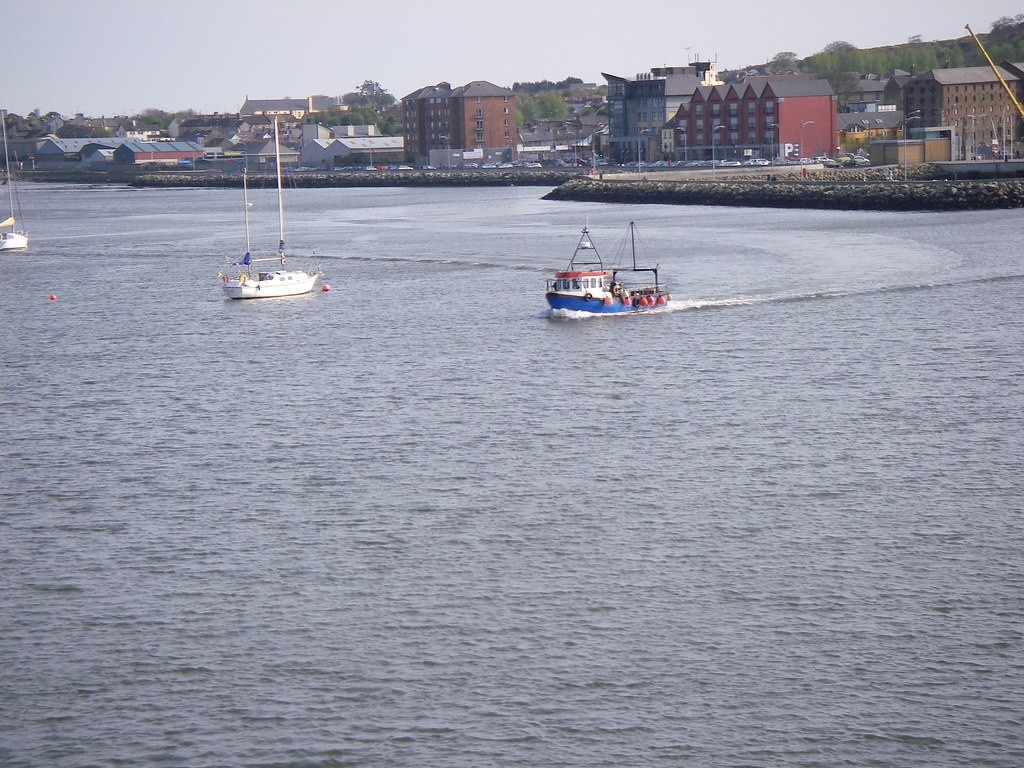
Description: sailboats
xmin=0 ymin=112 xmax=30 ymax=251
xmin=222 ymin=115 xmax=320 ymax=298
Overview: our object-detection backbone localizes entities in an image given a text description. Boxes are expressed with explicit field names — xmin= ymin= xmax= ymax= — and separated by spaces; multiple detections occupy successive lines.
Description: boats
xmin=543 ymin=220 xmax=671 ymax=312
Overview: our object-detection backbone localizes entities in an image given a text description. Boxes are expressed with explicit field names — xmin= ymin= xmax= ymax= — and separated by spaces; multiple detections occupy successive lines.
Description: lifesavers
xmin=223 ymin=275 xmax=229 ymax=282
xmin=634 ymin=297 xmax=640 ymax=307
xmin=239 ymin=274 xmax=247 ymax=283
xmin=586 ymin=293 xmax=592 ymax=301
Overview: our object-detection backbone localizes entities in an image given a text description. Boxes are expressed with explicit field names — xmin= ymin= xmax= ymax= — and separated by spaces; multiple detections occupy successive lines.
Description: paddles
xmin=0 ymin=217 xmax=15 ymax=227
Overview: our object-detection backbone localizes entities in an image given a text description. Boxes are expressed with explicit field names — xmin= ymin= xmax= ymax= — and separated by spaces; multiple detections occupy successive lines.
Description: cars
xmin=418 ymin=164 xmax=437 ymax=170
xmin=463 ymin=162 xmax=542 ymax=168
xmin=556 ymin=158 xmax=609 ymax=167
xmin=280 ymin=166 xmax=317 ymax=172
xmin=626 ymin=152 xmax=869 ymax=168
xmin=331 ymin=165 xmax=414 ymax=171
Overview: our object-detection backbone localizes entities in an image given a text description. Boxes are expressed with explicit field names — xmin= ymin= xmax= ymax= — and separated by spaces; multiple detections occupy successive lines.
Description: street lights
xmin=712 ymin=126 xmax=726 ymax=180
xmin=676 ymin=127 xmax=688 ymax=164
xmin=801 ymin=121 xmax=816 ymax=179
xmin=637 ymin=129 xmax=650 ymax=178
xmin=440 ymin=136 xmax=450 ymax=169
xmin=771 ymin=123 xmax=781 ymax=171
xmin=904 ymin=117 xmax=921 ymax=179
